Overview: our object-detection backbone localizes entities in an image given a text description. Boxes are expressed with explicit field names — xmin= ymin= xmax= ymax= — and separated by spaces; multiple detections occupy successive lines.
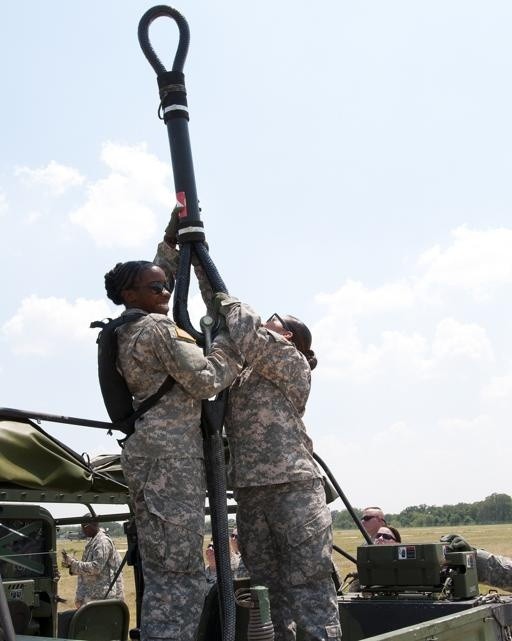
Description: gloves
xmin=440 ymin=533 xmax=477 ymax=557
xmin=164 ymin=198 xmax=202 ymax=242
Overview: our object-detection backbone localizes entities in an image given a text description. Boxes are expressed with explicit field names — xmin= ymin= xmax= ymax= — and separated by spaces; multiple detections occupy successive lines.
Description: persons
xmin=348 ymin=505 xmax=388 ymax=593
xmin=225 ymin=525 xmax=251 ymax=580
xmin=440 ymin=533 xmax=512 ymax=594
xmin=201 ymin=539 xmax=223 ymax=584
xmin=370 ymin=524 xmax=403 ymax=547
xmin=62 ymin=512 xmax=126 ymax=610
xmin=94 ymin=202 xmax=248 ymax=639
xmin=175 ymin=237 xmax=345 ymax=641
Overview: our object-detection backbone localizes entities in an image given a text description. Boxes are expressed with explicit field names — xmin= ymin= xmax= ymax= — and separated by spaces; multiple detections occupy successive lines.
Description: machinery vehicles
xmin=0 ymin=406 xmax=512 ymax=641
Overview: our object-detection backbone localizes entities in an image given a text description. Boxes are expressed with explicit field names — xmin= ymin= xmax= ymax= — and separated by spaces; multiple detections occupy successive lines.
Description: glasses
xmin=362 ymin=515 xmax=375 ymax=520
xmin=148 ymin=280 xmax=171 ymax=292
xmin=82 ymin=524 xmax=90 ymax=528
xmin=266 ymin=312 xmax=289 ymax=332
xmin=230 ymin=533 xmax=239 ymax=538
xmin=376 ymin=532 xmax=395 ymax=540
xmin=207 ymin=544 xmax=214 ymax=549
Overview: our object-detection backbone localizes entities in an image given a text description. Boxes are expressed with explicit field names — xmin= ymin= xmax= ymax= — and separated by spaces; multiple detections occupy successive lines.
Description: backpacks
xmin=89 ymin=312 xmax=176 ymax=447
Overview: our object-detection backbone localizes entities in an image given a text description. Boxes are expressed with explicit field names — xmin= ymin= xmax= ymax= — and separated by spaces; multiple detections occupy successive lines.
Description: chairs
xmin=68 ymin=599 xmax=132 ymax=640
